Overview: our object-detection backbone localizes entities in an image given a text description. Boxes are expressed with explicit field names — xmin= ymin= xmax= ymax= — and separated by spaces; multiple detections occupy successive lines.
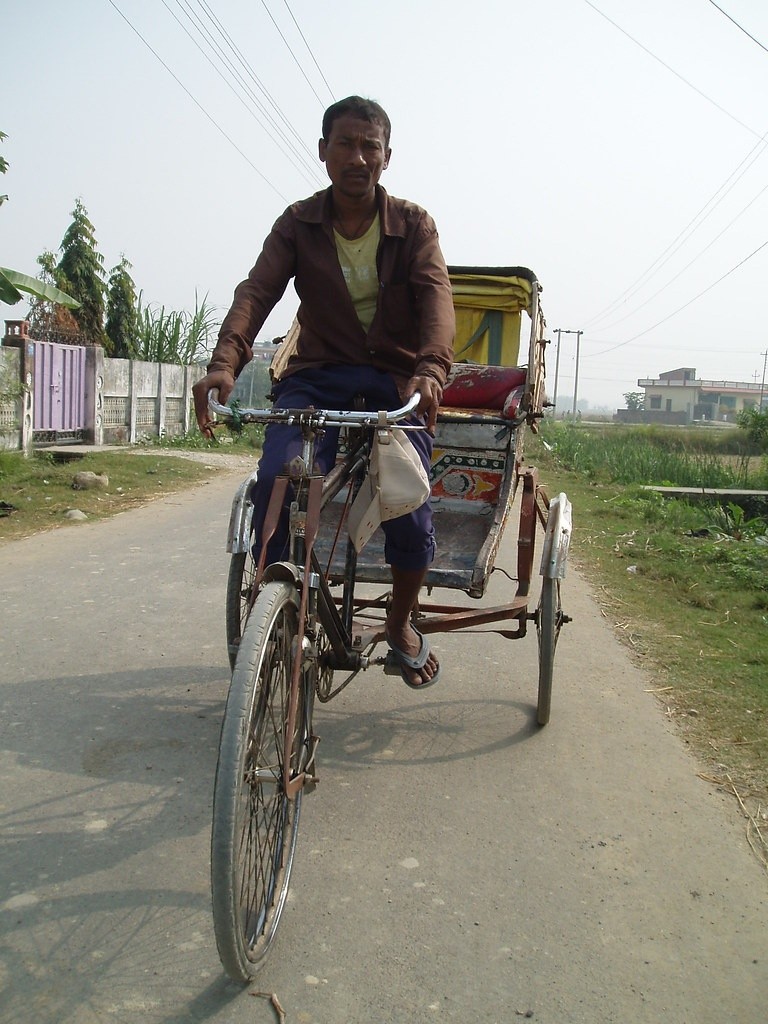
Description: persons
xmin=192 ymin=96 xmax=456 ymax=689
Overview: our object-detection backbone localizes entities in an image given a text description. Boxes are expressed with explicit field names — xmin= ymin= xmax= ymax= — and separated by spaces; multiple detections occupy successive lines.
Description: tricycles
xmin=202 ymin=263 xmax=574 ymax=981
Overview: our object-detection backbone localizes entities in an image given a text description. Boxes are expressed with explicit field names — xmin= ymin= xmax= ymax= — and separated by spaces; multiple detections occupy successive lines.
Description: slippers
xmin=385 ymin=615 xmax=441 ymax=689
xmin=230 ymin=628 xmax=277 ymax=655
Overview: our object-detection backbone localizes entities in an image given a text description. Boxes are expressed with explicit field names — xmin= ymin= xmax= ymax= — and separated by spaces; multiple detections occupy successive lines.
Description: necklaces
xmin=334 ymin=210 xmax=371 ymax=240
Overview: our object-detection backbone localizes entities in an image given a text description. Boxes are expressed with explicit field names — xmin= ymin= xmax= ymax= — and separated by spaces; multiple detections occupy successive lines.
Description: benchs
xmin=437 ymin=362 xmax=527 ymax=428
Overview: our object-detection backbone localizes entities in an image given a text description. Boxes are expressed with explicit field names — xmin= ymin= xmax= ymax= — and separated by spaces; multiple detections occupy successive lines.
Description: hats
xmin=345 ymin=410 xmax=432 ymax=552
